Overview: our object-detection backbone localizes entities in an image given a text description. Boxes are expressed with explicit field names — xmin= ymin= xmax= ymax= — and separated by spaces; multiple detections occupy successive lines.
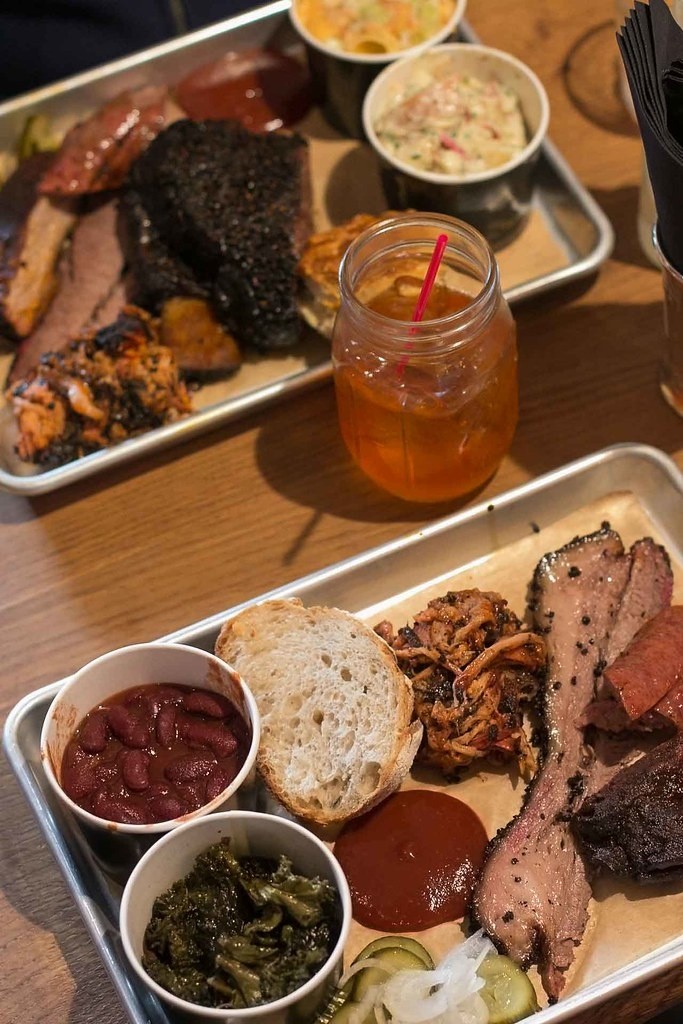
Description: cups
xmin=651 ymin=223 xmax=683 ymax=417
xmin=362 ymin=44 xmax=552 ymax=244
xmin=118 ymin=810 xmax=352 ymax=1024
xmin=38 ymin=642 xmax=261 ymax=888
xmin=287 ymin=0 xmax=469 ymax=139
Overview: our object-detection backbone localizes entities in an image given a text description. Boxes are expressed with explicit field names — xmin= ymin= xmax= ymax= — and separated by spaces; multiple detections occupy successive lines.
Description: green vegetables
xmin=142 ymin=835 xmax=339 ymax=1007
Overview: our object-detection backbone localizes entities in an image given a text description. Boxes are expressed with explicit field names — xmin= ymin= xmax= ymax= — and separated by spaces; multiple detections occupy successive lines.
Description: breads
xmin=214 ymin=597 xmax=423 ymax=825
xmin=294 ymin=208 xmax=447 ymax=340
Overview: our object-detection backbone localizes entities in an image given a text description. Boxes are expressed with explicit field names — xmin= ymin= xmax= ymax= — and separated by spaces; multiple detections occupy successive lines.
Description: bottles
xmin=330 ymin=211 xmax=519 ymax=505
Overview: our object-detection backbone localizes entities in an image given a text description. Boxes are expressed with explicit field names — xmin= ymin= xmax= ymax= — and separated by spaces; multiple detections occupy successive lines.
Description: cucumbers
xmin=311 ymin=935 xmax=537 ymax=1024
